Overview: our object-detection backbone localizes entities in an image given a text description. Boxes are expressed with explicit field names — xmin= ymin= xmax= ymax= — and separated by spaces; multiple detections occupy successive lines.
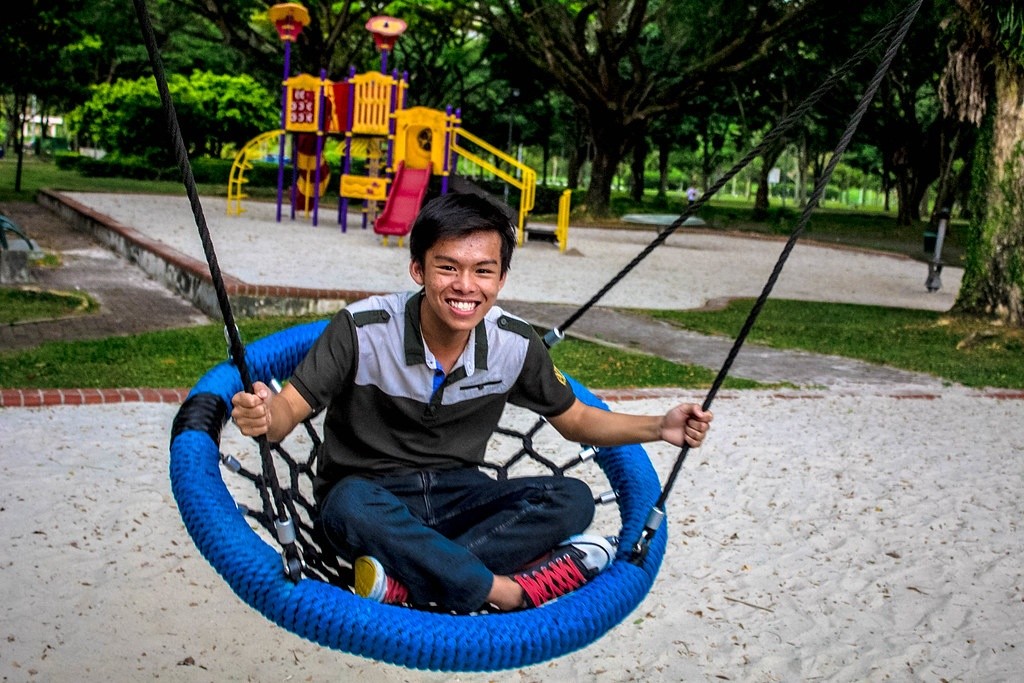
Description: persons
xmin=687 ymin=186 xmax=698 ymax=205
xmin=230 ymin=193 xmax=713 ymax=615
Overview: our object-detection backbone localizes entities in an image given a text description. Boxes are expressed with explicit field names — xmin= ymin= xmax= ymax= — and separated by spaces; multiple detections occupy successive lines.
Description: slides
xmin=287 ymin=133 xmax=331 ymax=212
xmin=373 ymin=161 xmax=432 ymax=236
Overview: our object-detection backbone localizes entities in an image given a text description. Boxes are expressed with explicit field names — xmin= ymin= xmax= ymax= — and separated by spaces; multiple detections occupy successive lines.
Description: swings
xmin=129 ymin=1 xmax=925 ymax=675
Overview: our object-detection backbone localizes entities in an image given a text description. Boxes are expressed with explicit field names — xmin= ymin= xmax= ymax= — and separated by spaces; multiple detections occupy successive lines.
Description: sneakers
xmin=354 ymin=556 xmax=413 ymax=608
xmin=489 ymin=535 xmax=615 ymax=612
